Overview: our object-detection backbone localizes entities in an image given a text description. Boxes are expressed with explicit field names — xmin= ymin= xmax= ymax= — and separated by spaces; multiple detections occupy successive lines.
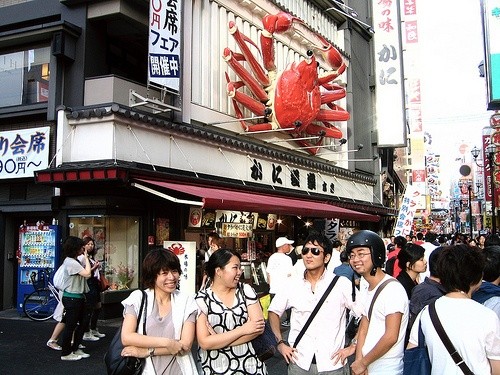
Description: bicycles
xmin=22 ymin=266 xmax=91 ymax=325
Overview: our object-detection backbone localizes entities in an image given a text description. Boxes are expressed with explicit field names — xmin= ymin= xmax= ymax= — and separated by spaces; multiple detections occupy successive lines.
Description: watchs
xmin=148 ymin=347 xmax=155 ymax=357
xmin=351 ymin=338 xmax=357 ymax=344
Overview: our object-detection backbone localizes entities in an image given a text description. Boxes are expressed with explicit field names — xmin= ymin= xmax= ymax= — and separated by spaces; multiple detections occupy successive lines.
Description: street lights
xmin=471 ymin=145 xmax=496 ymax=236
xmin=458 ymin=180 xmax=482 ymax=238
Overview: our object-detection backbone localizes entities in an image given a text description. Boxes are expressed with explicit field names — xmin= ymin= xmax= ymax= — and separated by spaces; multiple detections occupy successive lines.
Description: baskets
xmin=31 ymin=271 xmax=46 ymax=288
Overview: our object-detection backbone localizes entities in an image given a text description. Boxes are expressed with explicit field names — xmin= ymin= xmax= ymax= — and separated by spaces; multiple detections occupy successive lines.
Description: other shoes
xmin=284 ymin=323 xmax=290 ymax=326
xmin=281 ymin=321 xmax=288 ymax=325
xmin=71 ymin=342 xmax=86 ymax=348
xmin=47 ymin=339 xmax=62 ymax=350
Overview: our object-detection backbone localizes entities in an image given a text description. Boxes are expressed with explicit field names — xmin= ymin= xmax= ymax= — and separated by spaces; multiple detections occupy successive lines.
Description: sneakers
xmin=83 ymin=329 xmax=99 ymax=341
xmin=61 ymin=352 xmax=81 ymax=360
xmin=91 ymin=330 xmax=105 ymax=337
xmin=71 ymin=349 xmax=89 ymax=358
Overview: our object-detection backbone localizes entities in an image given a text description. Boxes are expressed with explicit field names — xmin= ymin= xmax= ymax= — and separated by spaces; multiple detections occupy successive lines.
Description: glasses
xmin=302 ymin=248 xmax=327 ymax=255
xmin=348 ymin=253 xmax=371 ymax=260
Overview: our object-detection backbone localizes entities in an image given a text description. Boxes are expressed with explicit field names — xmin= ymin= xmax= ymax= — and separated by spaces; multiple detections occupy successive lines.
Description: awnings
xmin=132 ymin=175 xmax=380 ymax=222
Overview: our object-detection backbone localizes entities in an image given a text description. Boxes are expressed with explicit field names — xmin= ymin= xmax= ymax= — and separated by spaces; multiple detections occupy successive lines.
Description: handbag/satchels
xmin=251 ymin=318 xmax=277 ymax=362
xmin=403 ymin=307 xmax=430 ymax=375
xmin=53 ymin=265 xmax=64 ymax=289
xmin=94 ymin=279 xmax=102 ymax=292
xmin=104 ymin=326 xmax=144 ymax=375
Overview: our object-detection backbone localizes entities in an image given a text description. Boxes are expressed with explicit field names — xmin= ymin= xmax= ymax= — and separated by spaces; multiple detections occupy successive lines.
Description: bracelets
xmin=276 ymin=340 xmax=284 ymax=345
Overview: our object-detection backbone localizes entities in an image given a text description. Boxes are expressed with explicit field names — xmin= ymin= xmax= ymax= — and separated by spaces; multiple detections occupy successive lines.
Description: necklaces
xmin=458 ymin=290 xmax=469 ymax=298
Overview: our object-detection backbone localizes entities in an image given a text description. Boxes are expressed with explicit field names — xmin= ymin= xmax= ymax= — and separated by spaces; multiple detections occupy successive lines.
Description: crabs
xmin=218 ymin=11 xmax=350 ymax=157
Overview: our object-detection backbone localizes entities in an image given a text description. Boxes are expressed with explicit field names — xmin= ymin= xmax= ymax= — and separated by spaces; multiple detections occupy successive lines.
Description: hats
xmin=276 ymin=237 xmax=294 ymax=247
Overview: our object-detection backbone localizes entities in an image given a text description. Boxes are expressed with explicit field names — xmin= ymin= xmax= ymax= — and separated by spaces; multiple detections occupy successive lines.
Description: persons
xmin=47 ymin=235 xmax=105 ymax=360
xmin=121 ymin=247 xmax=198 ymax=375
xmin=197 ymin=230 xmax=500 ymax=375
xmin=194 ymin=248 xmax=269 ymax=375
xmin=406 ymin=244 xmax=500 ymax=375
xmin=345 ymin=230 xmax=409 ymax=375
xmin=268 ymin=233 xmax=363 ymax=375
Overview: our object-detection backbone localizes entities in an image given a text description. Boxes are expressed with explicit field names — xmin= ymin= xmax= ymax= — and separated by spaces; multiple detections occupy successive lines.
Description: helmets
xmin=346 ymin=230 xmax=385 ymax=267
